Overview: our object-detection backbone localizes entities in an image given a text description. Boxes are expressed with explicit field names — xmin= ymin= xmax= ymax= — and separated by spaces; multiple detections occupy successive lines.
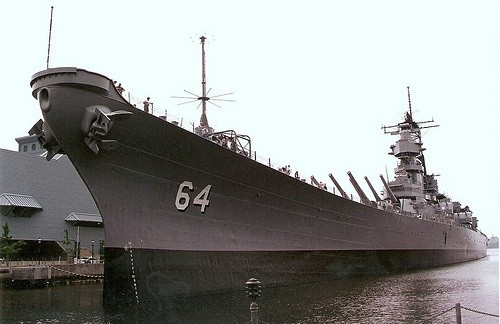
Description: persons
xmin=113 ymin=80 xmax=117 ymax=84
xmin=143 ymin=100 xmax=149 ymax=113
xmin=116 ymin=83 xmax=125 ymax=95
xmin=287 ymin=164 xmax=291 ymax=175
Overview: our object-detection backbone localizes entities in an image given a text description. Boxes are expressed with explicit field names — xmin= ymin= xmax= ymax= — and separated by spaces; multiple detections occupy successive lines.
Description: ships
xmin=28 ymin=67 xmax=490 ymax=304
